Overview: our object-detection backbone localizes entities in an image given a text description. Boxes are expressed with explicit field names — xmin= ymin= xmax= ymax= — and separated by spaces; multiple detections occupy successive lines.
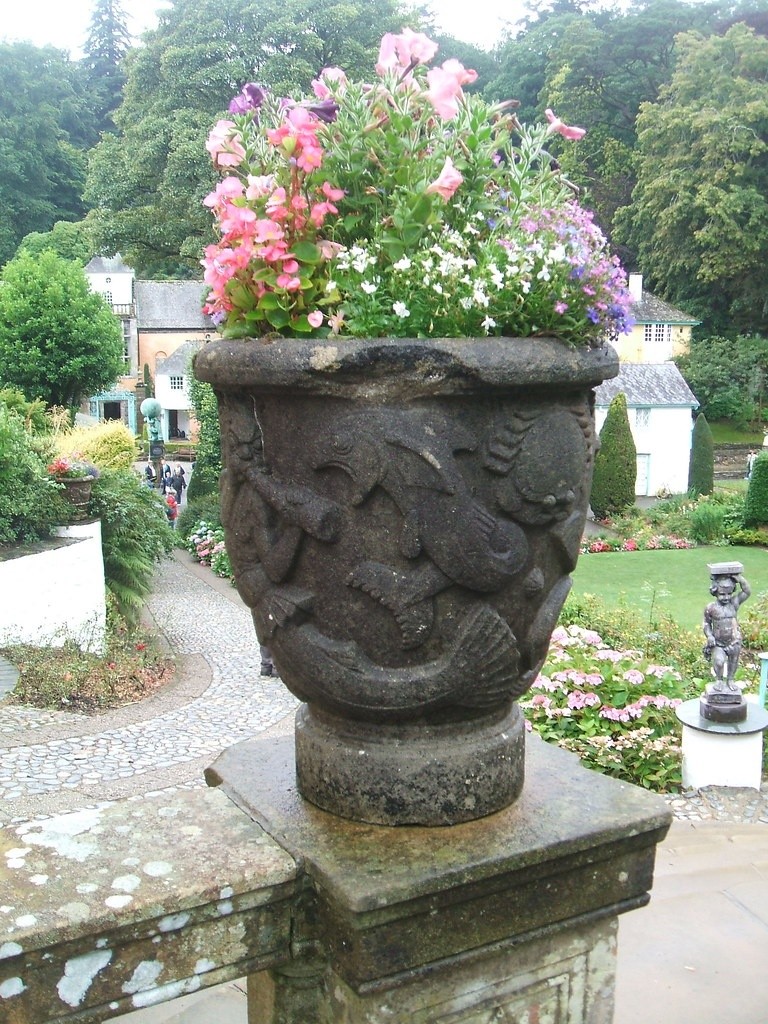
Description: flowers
xmin=203 ymin=26 xmax=637 ymax=354
xmin=47 ymin=451 xmax=100 ymax=479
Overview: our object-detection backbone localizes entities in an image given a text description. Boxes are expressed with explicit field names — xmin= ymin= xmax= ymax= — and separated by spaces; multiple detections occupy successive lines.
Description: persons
xmin=166 ymin=489 xmax=179 ymax=531
xmin=260 ymin=657 xmax=278 ymax=678
xmin=169 ymin=424 xmax=185 ymax=438
xmin=192 ymin=462 xmax=198 ymax=470
xmin=702 ymin=573 xmax=751 ymax=692
xmin=145 ymin=460 xmax=157 ymax=489
xmin=172 ymin=469 xmax=187 ymax=504
xmin=173 ymin=463 xmax=185 ymax=476
xmin=161 ymin=472 xmax=173 ymax=495
xmin=159 ymin=459 xmax=171 ymax=495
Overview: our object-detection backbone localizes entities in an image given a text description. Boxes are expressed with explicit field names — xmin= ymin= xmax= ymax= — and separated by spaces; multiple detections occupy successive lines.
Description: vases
xmin=55 ymin=475 xmax=94 ymax=520
xmin=193 ymin=336 xmax=619 ymax=825
